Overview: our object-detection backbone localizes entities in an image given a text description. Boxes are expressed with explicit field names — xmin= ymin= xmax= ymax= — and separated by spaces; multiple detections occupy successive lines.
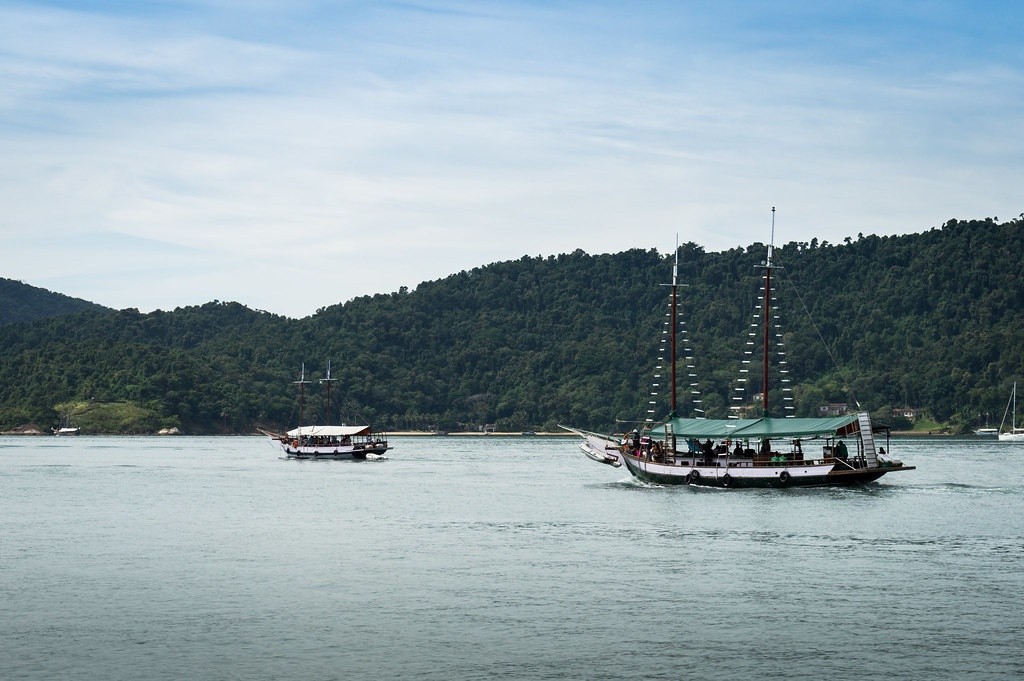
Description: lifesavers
xmin=297 ymin=450 xmax=301 ymax=456
xmin=722 ymin=474 xmax=731 ymax=487
xmin=780 ymin=471 xmax=791 ymax=483
xmin=684 ymin=470 xmax=698 ymax=485
xmin=334 ymin=450 xmax=338 ymax=456
xmin=287 ymin=448 xmax=290 ymax=454
xmin=314 ymin=451 xmax=319 ymax=457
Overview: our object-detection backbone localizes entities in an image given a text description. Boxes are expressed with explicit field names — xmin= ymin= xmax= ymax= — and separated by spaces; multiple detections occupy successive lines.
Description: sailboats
xmin=557 ymin=204 xmax=918 ymax=492
xmin=996 ymin=381 xmax=1024 ymax=443
xmin=255 ymin=356 xmax=394 ymax=461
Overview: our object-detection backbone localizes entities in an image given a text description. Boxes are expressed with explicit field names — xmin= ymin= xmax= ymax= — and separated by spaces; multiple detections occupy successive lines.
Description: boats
xmin=972 ymin=428 xmax=998 ymax=436
xmin=51 ymin=426 xmax=81 ymax=436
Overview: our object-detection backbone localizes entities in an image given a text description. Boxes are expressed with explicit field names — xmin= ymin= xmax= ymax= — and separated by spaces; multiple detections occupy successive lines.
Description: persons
xmin=836 ymin=441 xmax=848 ymax=458
xmin=292 ymin=436 xmax=351 ymax=449
xmin=632 ymin=429 xmax=640 ymax=456
xmin=368 ymin=435 xmax=374 ymax=446
xmin=761 ymin=441 xmax=770 ymax=452
xmin=733 ymin=442 xmax=743 ymax=455
xmin=879 ymin=447 xmax=885 ymax=454
xmin=705 ymin=443 xmax=716 ymax=466
xmin=645 ymin=437 xmax=663 ymax=454
